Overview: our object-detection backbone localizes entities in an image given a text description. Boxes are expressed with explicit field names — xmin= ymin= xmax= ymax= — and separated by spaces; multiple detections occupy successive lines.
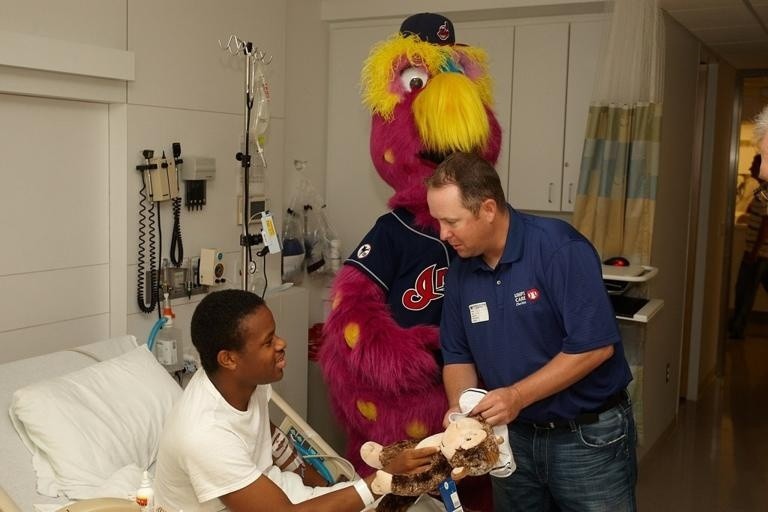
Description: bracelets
xmin=353 ymin=477 xmax=375 ymax=508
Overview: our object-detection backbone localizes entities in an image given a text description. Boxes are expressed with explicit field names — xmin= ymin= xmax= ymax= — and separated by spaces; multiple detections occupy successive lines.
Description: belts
xmin=518 ymin=390 xmax=627 ymax=432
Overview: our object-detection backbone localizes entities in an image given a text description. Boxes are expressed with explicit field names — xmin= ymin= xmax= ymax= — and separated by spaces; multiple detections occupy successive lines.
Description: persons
xmin=724 ymin=153 xmax=767 ymax=343
xmin=149 ymin=289 xmax=440 ymax=512
xmin=424 ymin=148 xmax=639 ymax=510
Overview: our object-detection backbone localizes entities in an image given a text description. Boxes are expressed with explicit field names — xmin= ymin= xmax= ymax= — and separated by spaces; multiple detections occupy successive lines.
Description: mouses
xmin=604 ymin=255 xmax=629 ymax=267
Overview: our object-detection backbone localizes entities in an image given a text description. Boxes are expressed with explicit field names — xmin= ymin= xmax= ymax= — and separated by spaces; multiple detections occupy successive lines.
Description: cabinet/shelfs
xmin=509 ymin=12 xmax=612 ymax=213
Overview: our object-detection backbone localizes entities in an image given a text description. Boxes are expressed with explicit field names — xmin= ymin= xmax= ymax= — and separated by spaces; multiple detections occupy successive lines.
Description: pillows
xmin=7 ymin=342 xmax=186 ymax=498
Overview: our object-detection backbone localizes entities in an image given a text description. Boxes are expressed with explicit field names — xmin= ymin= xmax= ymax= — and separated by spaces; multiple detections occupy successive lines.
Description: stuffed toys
xmin=319 ymin=11 xmax=505 ymax=477
xmin=358 ymin=415 xmax=504 ymax=499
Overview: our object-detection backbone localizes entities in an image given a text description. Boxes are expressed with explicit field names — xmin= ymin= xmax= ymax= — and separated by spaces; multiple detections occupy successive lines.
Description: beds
xmin=1 ymin=334 xmax=377 ymax=512
